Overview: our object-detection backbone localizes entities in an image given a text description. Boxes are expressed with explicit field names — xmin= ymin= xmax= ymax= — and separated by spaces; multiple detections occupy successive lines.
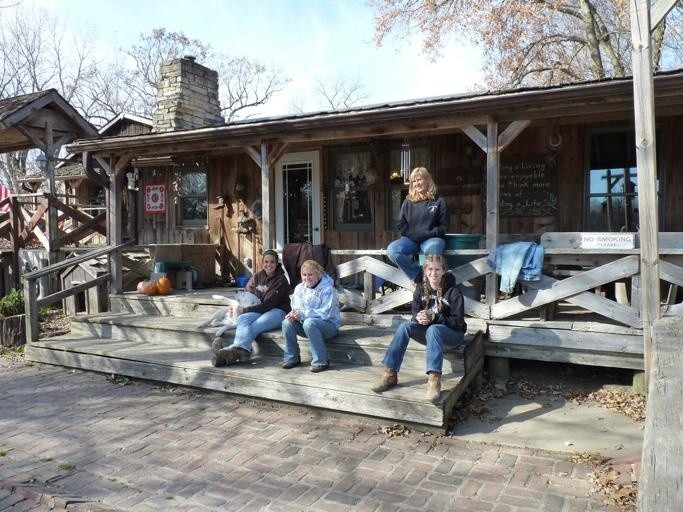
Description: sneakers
xmin=412 ymin=267 xmax=423 ymax=293
xmin=212 ymin=337 xmax=249 ymax=367
xmin=282 ymin=361 xmax=300 ymax=368
xmin=310 ymin=365 xmax=329 ymax=372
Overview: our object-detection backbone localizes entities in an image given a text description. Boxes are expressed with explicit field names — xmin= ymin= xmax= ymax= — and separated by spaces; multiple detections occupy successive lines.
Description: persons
xmin=370 ymin=254 xmax=467 ymax=402
xmin=281 ymin=260 xmax=340 ymax=373
xmin=386 ymin=167 xmax=450 ymax=288
xmin=211 ymin=250 xmax=292 ymax=367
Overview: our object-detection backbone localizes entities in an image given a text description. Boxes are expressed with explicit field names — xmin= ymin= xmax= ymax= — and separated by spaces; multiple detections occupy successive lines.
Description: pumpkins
xmin=136 ymin=275 xmax=171 ymax=296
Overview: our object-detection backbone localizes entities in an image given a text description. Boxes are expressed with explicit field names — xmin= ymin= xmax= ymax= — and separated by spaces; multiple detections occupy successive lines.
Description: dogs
xmin=197 ymin=285 xmax=269 ymax=337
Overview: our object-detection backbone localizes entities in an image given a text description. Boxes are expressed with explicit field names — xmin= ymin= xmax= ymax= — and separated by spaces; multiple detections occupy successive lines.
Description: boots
xmin=370 ymin=368 xmax=397 ymax=391
xmin=427 ymin=372 xmax=441 ymax=401
xmin=213 ymin=198 xmax=224 ymax=209
xmin=232 ymin=210 xmax=253 ymax=233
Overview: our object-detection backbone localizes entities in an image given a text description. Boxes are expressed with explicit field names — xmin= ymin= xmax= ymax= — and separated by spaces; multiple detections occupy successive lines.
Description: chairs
xmin=381 ymin=247 xmax=398 ymax=295
